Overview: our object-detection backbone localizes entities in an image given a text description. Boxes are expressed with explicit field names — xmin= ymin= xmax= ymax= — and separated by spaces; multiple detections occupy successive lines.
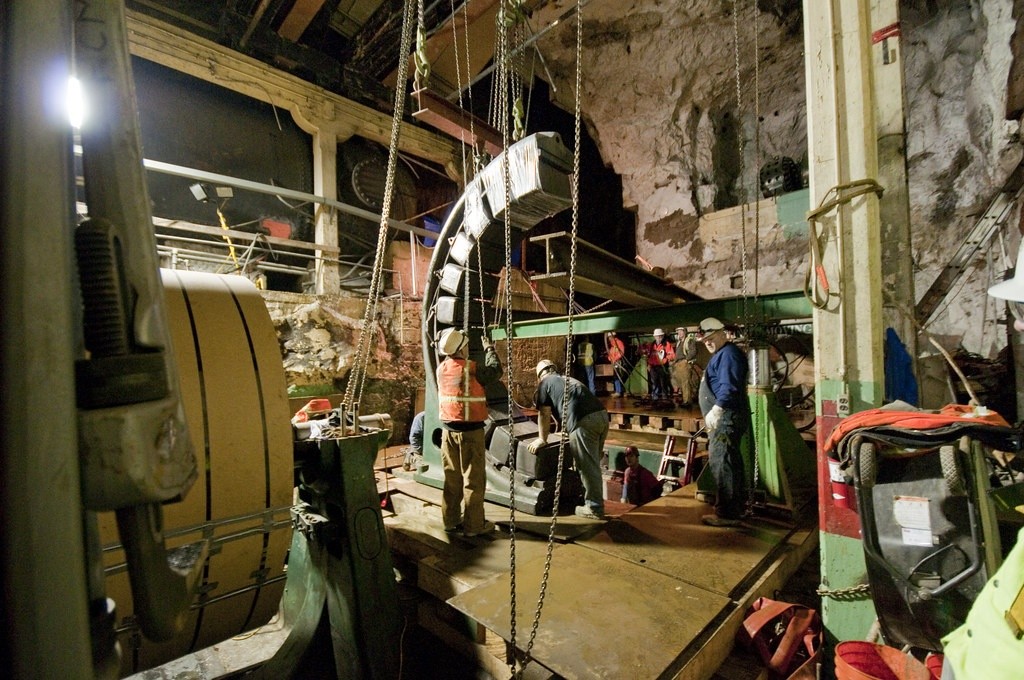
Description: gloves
xmin=526 ymin=438 xmax=548 ymax=455
xmin=704 ymin=405 xmax=726 ymax=430
xmin=480 ymin=335 xmax=494 ymax=352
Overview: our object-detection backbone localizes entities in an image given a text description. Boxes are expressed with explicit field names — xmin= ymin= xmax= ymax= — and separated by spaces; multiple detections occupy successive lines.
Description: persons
xmin=619 ymin=446 xmax=664 ymax=504
xmin=695 ymin=317 xmax=751 ymax=526
xmin=576 ymin=332 xmax=625 ymax=396
xmin=526 ymin=359 xmax=609 ymax=520
xmin=436 ymin=328 xmax=504 ymax=537
xmin=646 ymin=327 xmax=696 ymax=409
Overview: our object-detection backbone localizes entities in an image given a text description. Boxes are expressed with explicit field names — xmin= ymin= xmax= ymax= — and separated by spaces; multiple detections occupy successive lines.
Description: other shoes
xmin=444 ymin=522 xmax=463 ymax=535
xmin=463 ymin=521 xmax=495 ymax=537
xmin=610 ymin=393 xmax=623 ymax=398
xmin=402 ymin=453 xmax=411 ymax=471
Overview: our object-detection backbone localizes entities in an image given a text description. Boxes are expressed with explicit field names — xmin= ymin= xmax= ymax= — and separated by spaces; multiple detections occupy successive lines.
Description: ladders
xmin=654 ymin=435 xmax=698 ymax=490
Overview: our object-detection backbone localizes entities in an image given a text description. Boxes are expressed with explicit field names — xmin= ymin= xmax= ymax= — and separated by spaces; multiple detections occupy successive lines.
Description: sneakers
xmin=574 ymin=504 xmax=604 ymax=520
xmin=701 ymin=514 xmax=737 ymax=526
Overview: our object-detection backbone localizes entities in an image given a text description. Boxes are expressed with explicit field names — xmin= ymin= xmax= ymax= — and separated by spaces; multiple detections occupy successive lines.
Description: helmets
xmin=695 ymin=317 xmax=725 ymax=342
xmin=536 ymin=359 xmax=557 ymax=379
xmin=624 ymin=446 xmax=640 ymax=456
xmin=436 ymin=327 xmax=469 ymax=356
xmin=607 ymin=331 xmax=617 ymax=337
xmin=674 ymin=326 xmax=687 ymax=334
xmin=652 ymin=328 xmax=665 ymax=337
